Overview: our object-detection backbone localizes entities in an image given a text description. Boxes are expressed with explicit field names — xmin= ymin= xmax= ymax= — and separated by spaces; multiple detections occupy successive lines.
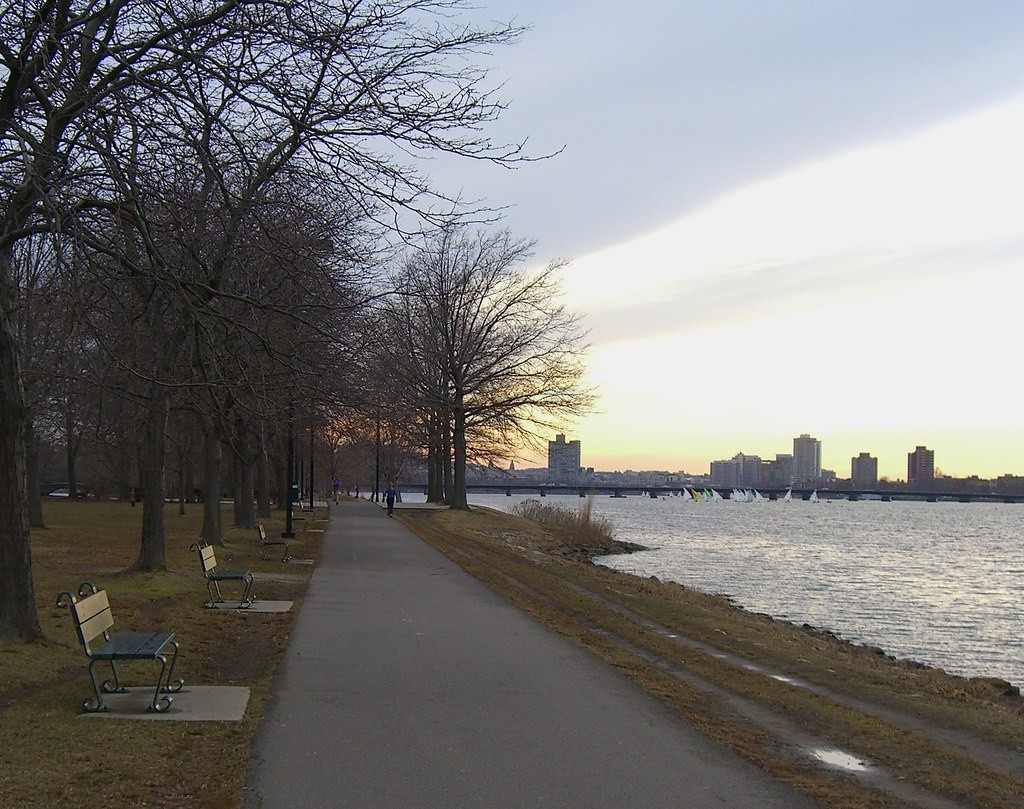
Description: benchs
xmin=184 ymin=539 xmax=257 ymax=608
xmin=291 ymin=508 xmax=306 ymax=532
xmin=299 ymin=501 xmax=314 ymax=518
xmin=56 ymin=582 xmax=184 ymax=712
xmin=256 ymin=522 xmax=291 ymax=563
